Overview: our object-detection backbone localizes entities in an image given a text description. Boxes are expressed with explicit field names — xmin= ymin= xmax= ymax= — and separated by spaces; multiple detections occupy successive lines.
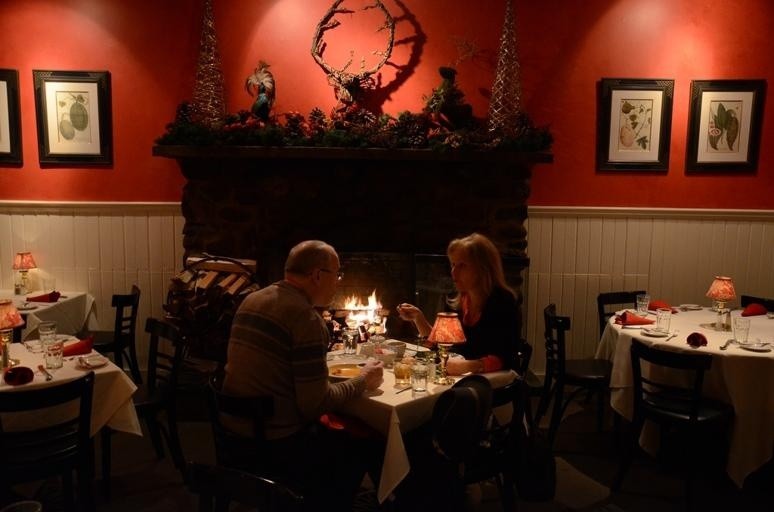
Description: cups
xmin=655 ymin=307 xmax=672 ymax=333
xmin=635 ymin=295 xmax=650 ymax=315
xmin=341 ymin=326 xmax=358 ymax=354
xmin=734 ymin=318 xmax=749 ymax=344
xmin=360 ymin=335 xmax=396 ymax=369
xmin=38 ymin=322 xmax=62 ymax=368
xmin=387 ymin=342 xmax=406 ymax=362
xmin=393 ymin=357 xmax=435 ymax=392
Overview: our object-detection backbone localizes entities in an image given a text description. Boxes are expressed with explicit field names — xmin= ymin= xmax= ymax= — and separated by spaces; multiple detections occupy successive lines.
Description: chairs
xmin=100 ymin=317 xmax=192 ymax=483
xmin=532 ymin=302 xmax=613 ymax=446
xmin=742 ymin=295 xmax=773 ymax=311
xmin=208 ymin=277 xmax=556 ymax=512
xmin=78 ymin=286 xmax=143 ymax=384
xmin=1 ymin=371 xmax=96 ymax=512
xmin=597 ymin=292 xmax=647 ymax=339
xmin=628 ymin=337 xmax=734 ymax=490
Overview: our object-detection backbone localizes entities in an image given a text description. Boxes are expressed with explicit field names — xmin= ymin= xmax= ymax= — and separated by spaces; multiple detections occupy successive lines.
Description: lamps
xmin=697 ymin=275 xmax=738 ymax=333
xmin=1 ymin=304 xmax=25 ymax=368
xmin=11 ymin=250 xmax=38 ymax=296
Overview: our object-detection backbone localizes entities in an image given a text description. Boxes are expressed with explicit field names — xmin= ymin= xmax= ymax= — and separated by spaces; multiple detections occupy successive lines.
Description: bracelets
xmin=479 ymin=359 xmax=483 ymax=374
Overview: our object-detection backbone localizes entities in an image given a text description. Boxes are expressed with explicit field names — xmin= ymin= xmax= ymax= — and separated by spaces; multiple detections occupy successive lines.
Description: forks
xmin=664 ymin=329 xmax=680 ymax=342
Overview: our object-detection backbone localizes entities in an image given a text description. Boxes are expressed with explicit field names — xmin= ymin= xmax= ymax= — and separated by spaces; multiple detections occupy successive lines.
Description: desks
xmin=594 ymin=303 xmax=774 ymax=489
xmin=149 ymin=139 xmax=561 ymax=341
xmin=1 ymin=334 xmax=143 ymax=438
xmin=2 ymin=290 xmax=97 ymax=342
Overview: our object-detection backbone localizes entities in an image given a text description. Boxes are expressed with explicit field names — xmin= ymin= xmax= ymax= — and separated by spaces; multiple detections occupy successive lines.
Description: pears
xmin=621 ymin=119 xmax=633 ymax=147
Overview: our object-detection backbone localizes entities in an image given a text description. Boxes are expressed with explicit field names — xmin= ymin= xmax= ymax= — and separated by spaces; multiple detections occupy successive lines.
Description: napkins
xmin=687 ymin=332 xmax=708 ymax=347
xmin=63 ymin=335 xmax=93 ymax=357
xmin=615 ymin=311 xmax=654 ymax=325
xmin=649 ymin=300 xmax=678 ymax=313
xmin=27 ymin=291 xmax=60 ymax=302
xmin=4 ymin=367 xmax=34 ymax=385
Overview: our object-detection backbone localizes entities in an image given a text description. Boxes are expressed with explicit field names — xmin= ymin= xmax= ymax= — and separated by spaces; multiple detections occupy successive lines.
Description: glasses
xmin=321 ymin=267 xmax=345 ymax=281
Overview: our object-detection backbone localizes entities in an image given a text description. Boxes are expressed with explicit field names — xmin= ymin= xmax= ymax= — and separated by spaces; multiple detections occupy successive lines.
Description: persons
xmin=373 ymin=234 xmax=523 ymax=511
xmin=218 ymin=241 xmax=382 ymax=512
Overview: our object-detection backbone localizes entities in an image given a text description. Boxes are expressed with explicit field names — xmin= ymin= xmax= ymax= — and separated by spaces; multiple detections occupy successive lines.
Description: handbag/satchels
xmin=511 ymin=375 xmax=557 ymax=503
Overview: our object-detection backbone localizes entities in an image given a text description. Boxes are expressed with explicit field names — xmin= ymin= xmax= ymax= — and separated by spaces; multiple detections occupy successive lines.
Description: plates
xmin=326 ymin=361 xmax=362 ymax=379
xmin=75 ymin=357 xmax=108 ymax=369
xmin=740 ymin=345 xmax=770 ymax=351
xmin=680 ymin=303 xmax=703 ymax=310
xmin=15 ymin=303 xmax=38 ymax=310
xmin=639 ymin=327 xmax=668 ymax=337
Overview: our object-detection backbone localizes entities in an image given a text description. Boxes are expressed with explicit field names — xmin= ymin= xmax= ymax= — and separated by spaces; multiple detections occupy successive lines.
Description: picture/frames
xmin=30 ymin=67 xmax=115 ymax=169
xmin=0 ymin=67 xmax=24 ymax=170
xmin=594 ymin=76 xmax=676 ymax=173
xmin=686 ymin=77 xmax=768 ymax=177
